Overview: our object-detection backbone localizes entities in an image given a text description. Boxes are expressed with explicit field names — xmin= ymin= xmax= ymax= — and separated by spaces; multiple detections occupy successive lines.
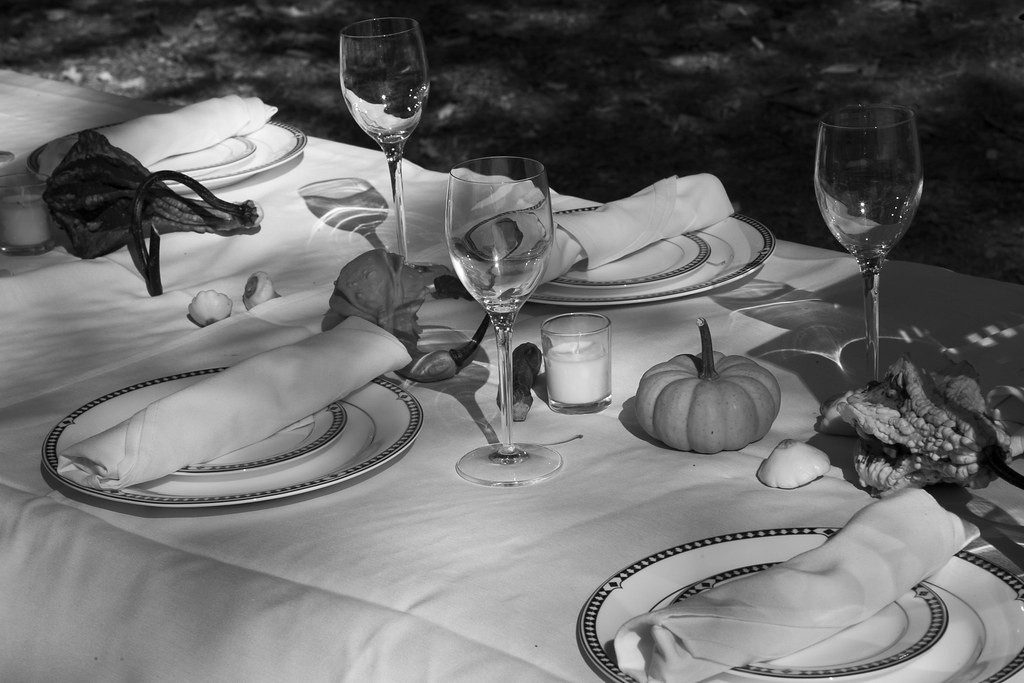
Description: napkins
xmin=38 ymin=94 xmax=278 ymax=181
xmin=612 ymin=486 xmax=982 ymax=683
xmin=54 ymin=314 xmax=413 ymax=491
xmin=535 ymin=170 xmax=735 ymax=290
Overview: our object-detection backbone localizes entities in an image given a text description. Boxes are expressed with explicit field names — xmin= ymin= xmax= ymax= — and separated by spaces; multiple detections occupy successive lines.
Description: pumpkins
xmin=634 ymin=317 xmax=780 ymax=454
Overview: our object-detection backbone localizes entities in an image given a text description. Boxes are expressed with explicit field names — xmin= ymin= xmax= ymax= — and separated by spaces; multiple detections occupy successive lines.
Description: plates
xmin=525 ymin=206 xmax=776 ymax=307
xmin=26 ymin=120 xmax=307 ymax=194
xmin=576 ymin=527 xmax=1024 ymax=683
xmin=38 ymin=364 xmax=424 ymax=509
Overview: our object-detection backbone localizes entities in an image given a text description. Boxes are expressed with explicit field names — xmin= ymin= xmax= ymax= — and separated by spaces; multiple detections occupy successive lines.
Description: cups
xmin=540 ymin=312 xmax=613 ymax=416
xmin=0 ymin=173 xmax=57 ymax=255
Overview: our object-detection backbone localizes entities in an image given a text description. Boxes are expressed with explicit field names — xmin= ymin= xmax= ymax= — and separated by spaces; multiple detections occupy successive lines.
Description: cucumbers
xmin=512 ymin=341 xmax=542 ymax=422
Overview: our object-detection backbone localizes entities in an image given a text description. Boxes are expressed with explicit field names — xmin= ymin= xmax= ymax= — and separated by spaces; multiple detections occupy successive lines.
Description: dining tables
xmin=0 ymin=62 xmax=1024 ymax=683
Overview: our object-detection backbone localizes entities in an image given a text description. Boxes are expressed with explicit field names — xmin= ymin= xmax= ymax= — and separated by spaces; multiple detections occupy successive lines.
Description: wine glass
xmin=338 ymin=16 xmax=430 ymax=264
xmin=444 ymin=155 xmax=563 ymax=488
xmin=813 ymin=104 xmax=923 ymax=392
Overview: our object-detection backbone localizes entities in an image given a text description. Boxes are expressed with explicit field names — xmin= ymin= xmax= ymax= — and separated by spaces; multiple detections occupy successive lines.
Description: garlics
xmin=242 ymin=271 xmax=281 ymax=311
xmin=188 ymin=290 xmax=233 ymax=326
